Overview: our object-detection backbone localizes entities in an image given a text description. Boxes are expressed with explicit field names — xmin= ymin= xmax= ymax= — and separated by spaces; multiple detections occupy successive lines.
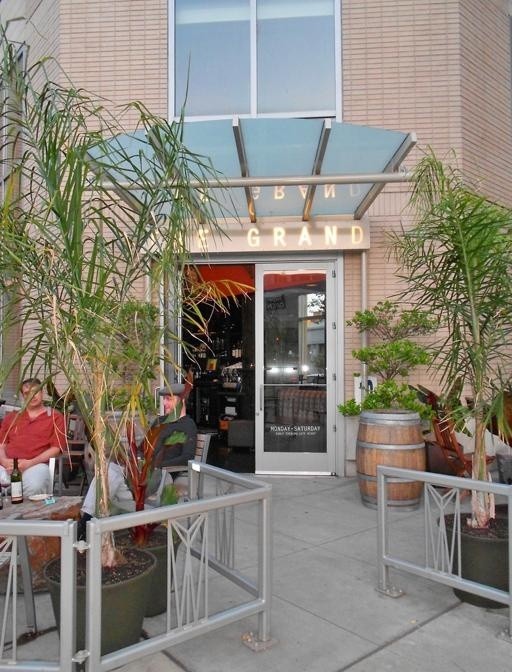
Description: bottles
xmin=0 ymin=481 xmax=4 ymax=509
xmin=186 ymin=318 xmax=243 ymax=382
xmin=10 ymin=457 xmax=23 ymax=504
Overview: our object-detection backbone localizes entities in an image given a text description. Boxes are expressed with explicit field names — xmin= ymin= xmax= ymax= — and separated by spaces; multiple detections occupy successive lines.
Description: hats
xmin=159 ymin=385 xmax=185 ymax=395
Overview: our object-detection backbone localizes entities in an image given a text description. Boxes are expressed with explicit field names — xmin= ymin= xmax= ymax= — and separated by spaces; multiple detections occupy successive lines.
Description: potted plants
xmin=384 ymin=139 xmax=512 ymax=611
xmin=0 ymin=15 xmax=244 ymax=659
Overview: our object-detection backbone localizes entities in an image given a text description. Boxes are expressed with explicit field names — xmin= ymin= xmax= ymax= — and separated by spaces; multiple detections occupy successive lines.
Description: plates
xmin=28 ymin=494 xmax=48 ymax=502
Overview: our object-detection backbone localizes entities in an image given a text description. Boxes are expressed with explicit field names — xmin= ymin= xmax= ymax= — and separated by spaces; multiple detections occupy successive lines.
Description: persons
xmin=0 ymin=378 xmax=66 ymax=497
xmin=77 ymin=384 xmax=197 ymax=542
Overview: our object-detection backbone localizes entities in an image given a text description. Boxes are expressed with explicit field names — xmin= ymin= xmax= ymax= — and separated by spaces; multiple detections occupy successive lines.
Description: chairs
xmin=431 ymin=416 xmax=499 ymax=505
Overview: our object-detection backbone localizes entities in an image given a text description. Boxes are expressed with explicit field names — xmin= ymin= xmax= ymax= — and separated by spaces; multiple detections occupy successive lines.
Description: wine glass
xmin=2 ymin=470 xmax=12 ymax=506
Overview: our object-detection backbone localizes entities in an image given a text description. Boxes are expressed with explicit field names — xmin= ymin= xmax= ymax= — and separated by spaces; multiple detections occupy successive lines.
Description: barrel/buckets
xmin=355 ymin=410 xmax=426 ymax=513
